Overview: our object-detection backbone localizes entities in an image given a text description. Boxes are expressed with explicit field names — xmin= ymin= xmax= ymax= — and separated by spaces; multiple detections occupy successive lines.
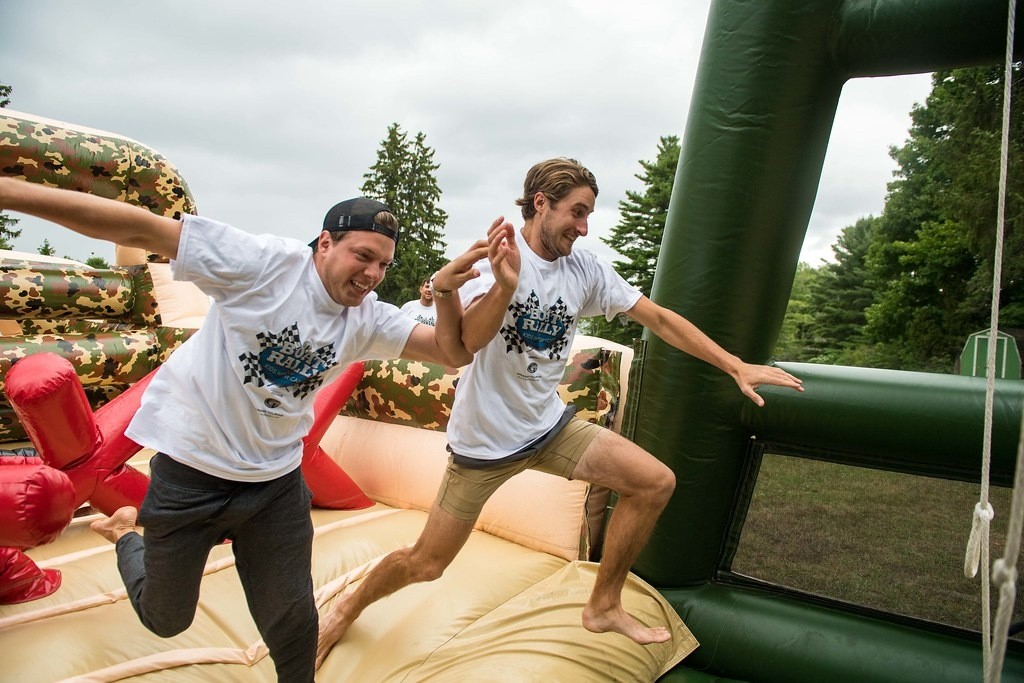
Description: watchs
xmin=429 ymin=270 xmax=457 ymax=297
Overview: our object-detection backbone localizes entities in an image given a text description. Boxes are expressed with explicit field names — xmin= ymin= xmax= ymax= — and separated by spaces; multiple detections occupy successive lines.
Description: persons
xmin=0 ymin=172 xmax=490 ymax=683
xmin=400 ymin=277 xmax=437 ymax=326
xmin=315 ymin=158 xmax=804 ymax=672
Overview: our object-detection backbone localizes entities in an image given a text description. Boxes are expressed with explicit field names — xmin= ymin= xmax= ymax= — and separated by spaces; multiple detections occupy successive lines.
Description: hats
xmin=308 ymin=198 xmax=399 ymax=251
